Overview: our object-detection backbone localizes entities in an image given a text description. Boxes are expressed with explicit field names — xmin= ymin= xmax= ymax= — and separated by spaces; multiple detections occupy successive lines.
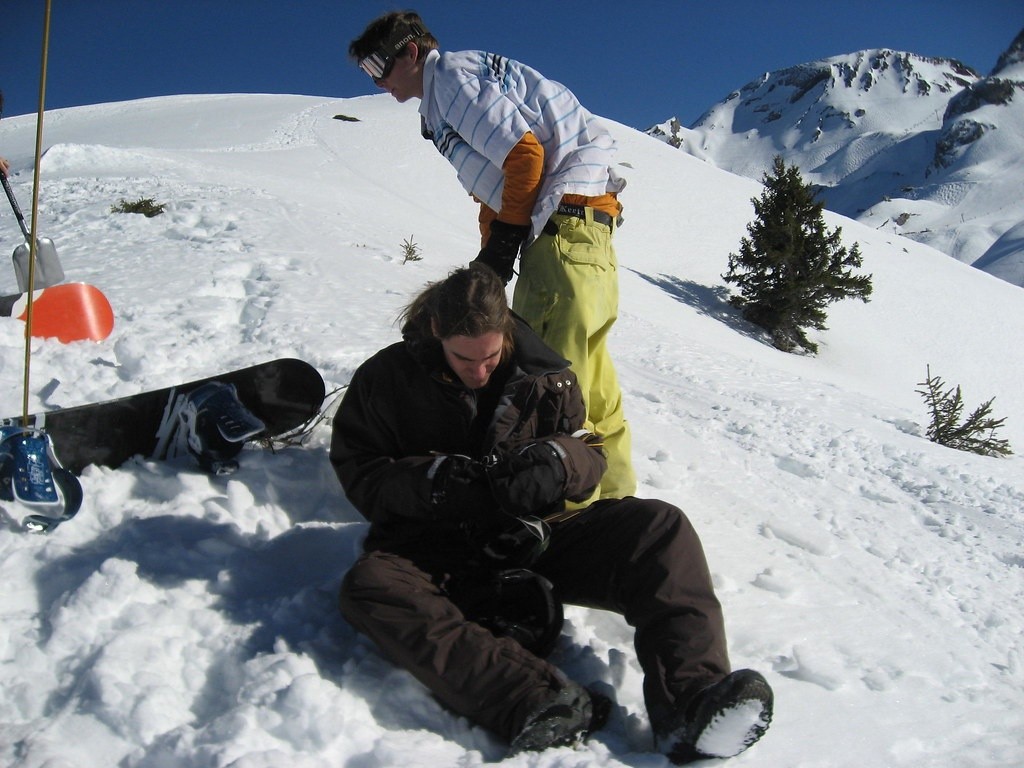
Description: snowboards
xmin=1 ymin=358 xmax=327 ymax=504
xmin=0 ymin=282 xmax=115 ymax=344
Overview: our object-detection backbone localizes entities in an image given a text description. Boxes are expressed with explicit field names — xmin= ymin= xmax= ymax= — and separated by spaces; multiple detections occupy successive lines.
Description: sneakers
xmin=653 ymin=669 xmax=771 ymax=766
xmin=507 ymin=684 xmax=612 ymax=759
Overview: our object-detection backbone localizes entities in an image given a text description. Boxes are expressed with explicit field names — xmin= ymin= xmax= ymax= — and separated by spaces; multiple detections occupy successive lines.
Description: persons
xmin=348 ymin=12 xmax=637 ymax=510
xmin=329 ymin=263 xmax=773 ymax=766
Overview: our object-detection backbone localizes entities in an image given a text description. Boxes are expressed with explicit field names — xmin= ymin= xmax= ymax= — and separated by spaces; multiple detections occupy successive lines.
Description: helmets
xmin=468 ymin=568 xmax=562 ymax=655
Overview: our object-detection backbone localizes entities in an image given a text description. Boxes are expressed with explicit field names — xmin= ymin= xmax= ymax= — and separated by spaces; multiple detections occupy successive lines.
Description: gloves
xmin=469 ymin=219 xmax=532 ymax=287
xmin=491 ymin=442 xmax=566 ymax=517
xmin=432 ymin=455 xmax=499 ymax=542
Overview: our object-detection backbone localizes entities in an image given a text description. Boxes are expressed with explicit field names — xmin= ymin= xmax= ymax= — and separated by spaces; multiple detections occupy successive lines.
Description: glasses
xmin=360 ymin=21 xmax=427 ymax=83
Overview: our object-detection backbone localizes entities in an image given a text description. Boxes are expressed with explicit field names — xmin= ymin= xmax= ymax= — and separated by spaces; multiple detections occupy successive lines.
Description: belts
xmin=541 ymin=202 xmax=625 ymax=236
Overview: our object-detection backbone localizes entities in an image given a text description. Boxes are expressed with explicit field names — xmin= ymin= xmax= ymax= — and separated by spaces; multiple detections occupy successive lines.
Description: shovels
xmin=0 ymin=169 xmax=67 ymax=292
